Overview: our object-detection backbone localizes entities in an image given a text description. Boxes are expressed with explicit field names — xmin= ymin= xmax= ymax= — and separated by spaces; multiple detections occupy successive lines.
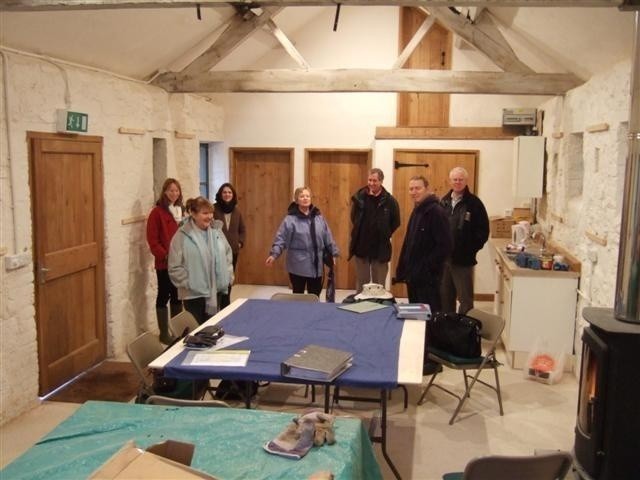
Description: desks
xmin=148 ymin=300 xmax=428 ymax=480
xmin=1 ymin=400 xmax=381 ymax=480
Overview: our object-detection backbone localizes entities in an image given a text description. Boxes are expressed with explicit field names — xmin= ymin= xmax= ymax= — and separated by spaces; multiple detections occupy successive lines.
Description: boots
xmin=170 ymin=303 xmax=183 ymax=318
xmin=157 ymin=306 xmax=176 ymax=346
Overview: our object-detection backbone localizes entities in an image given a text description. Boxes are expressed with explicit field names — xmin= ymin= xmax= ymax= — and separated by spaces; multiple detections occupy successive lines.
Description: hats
xmin=354 ymin=283 xmax=393 ymax=300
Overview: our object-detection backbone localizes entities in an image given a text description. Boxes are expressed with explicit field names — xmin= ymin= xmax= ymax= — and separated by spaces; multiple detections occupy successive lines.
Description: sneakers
xmin=423 ymin=362 xmax=442 ymax=375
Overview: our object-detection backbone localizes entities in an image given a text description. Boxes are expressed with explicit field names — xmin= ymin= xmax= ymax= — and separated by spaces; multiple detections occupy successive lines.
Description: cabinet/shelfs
xmin=512 ymin=136 xmax=546 ymax=198
xmin=494 ymin=242 xmax=577 ymax=375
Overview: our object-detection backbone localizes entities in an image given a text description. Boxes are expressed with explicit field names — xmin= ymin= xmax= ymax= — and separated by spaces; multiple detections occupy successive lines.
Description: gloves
xmin=183 ymin=333 xmax=217 ymax=347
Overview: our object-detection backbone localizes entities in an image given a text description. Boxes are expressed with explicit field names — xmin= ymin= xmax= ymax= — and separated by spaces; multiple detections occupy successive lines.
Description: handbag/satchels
xmin=430 ymin=312 xmax=481 ymax=358
xmin=215 ymin=380 xmax=258 ymax=401
xmin=153 ymin=327 xmax=209 ymax=400
xmin=326 ymin=272 xmax=335 ymax=303
xmin=344 ymin=296 xmax=396 ymax=307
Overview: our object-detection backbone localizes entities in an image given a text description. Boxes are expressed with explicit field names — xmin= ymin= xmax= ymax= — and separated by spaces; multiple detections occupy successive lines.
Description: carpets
xmin=42 ymin=361 xmax=141 ymax=402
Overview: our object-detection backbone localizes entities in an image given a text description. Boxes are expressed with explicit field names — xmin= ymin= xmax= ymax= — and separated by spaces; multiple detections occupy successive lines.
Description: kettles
xmin=511 ymin=225 xmax=529 ymax=246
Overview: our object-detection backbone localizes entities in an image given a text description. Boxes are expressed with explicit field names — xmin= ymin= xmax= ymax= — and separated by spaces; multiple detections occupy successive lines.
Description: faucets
xmin=530 ymin=231 xmax=547 ymax=251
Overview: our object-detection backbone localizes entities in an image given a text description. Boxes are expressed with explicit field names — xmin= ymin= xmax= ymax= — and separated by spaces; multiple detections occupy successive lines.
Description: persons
xmin=265 ymin=186 xmax=340 ymax=298
xmin=346 ymin=168 xmax=399 ymax=295
xmin=168 ymin=197 xmax=234 ymax=324
xmin=147 ymin=178 xmax=191 ymax=344
xmin=440 ymin=167 xmax=489 ymax=316
xmin=395 ymin=176 xmax=450 ymax=376
xmin=210 ymin=182 xmax=246 ymax=312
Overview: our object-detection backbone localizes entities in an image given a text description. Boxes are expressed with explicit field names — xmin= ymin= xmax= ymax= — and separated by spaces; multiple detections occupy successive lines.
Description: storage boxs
xmin=503 ymin=108 xmax=536 ymax=126
xmin=513 ymin=207 xmax=531 ymax=221
xmin=490 ymin=218 xmax=515 ymax=238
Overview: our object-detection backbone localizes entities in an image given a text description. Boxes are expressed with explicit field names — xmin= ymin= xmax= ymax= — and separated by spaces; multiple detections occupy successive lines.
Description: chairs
xmin=271 ymin=292 xmax=318 ymax=301
xmin=443 ymin=451 xmax=571 ymax=480
xmin=145 ymin=395 xmax=230 ymax=407
xmin=168 ymin=312 xmax=200 ymax=341
xmin=129 ymin=331 xmax=217 ymax=405
xmin=417 ymin=308 xmax=505 ymax=425
xmin=342 ymin=295 xmax=396 ymax=305
